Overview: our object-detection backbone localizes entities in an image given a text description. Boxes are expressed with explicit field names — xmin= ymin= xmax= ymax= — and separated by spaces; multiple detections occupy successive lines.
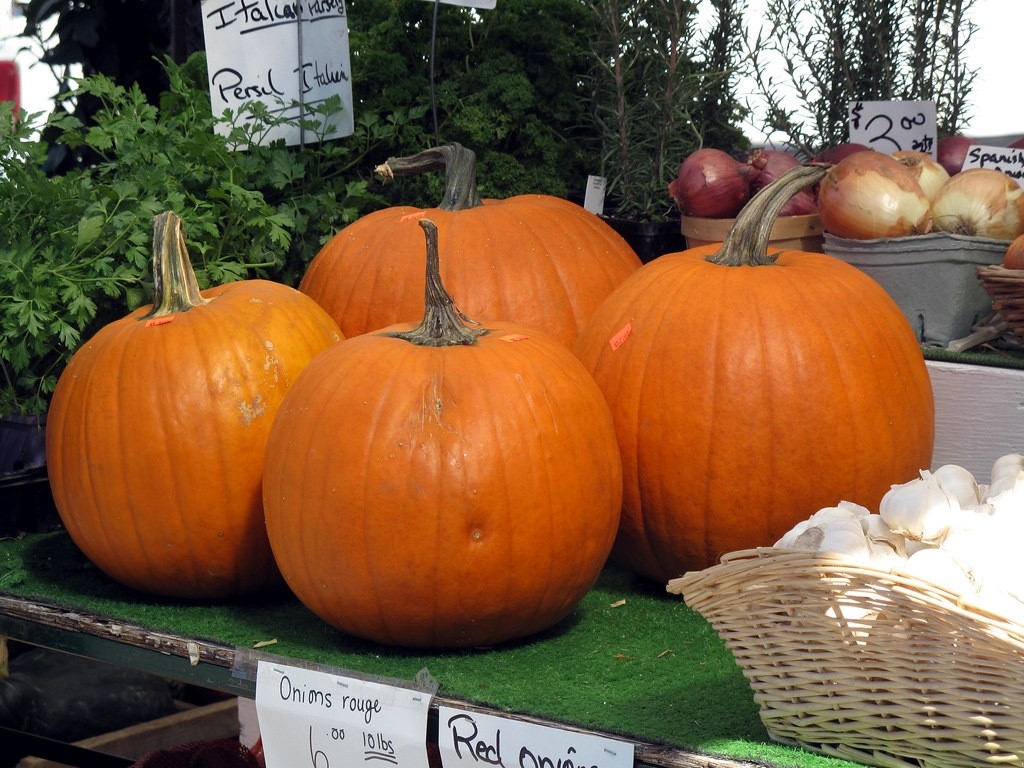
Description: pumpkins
xmin=46 ymin=142 xmax=934 ymax=650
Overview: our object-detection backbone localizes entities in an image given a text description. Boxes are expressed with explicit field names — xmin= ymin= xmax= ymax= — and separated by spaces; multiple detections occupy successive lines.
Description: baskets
xmin=666 ymin=547 xmax=1024 ymax=768
xmin=977 ymin=264 xmax=1024 ymax=336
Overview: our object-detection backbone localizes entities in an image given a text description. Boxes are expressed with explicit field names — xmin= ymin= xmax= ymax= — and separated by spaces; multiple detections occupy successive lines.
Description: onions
xmin=668 ymin=136 xmax=1024 ymax=269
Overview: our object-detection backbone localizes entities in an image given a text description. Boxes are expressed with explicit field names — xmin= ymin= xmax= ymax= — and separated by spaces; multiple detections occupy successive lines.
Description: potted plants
xmin=575 ymin=0 xmax=748 ymax=263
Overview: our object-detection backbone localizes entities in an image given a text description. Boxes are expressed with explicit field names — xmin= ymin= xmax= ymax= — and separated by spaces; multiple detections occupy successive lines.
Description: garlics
xmin=771 ymin=454 xmax=1024 ymax=648
